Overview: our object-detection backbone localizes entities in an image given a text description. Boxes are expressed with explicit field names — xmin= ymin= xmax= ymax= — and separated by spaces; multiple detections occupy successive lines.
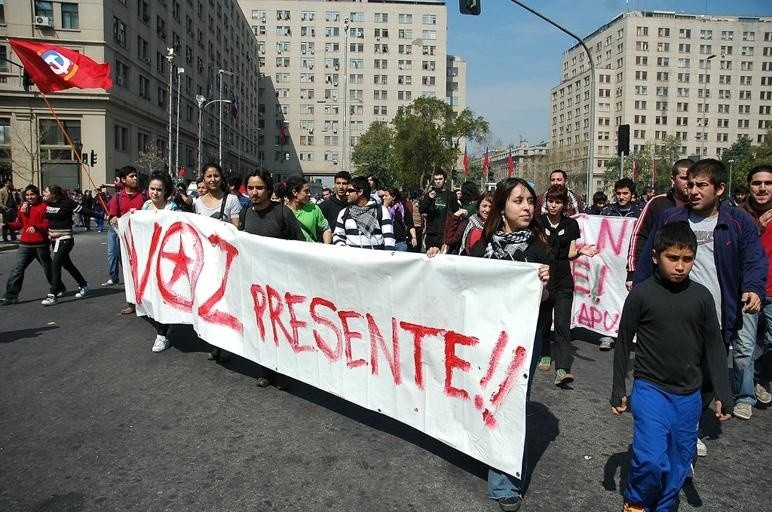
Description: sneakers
xmin=538 ymin=357 xmax=551 ymax=370
xmin=732 ymin=403 xmax=753 ymax=419
xmin=555 ymin=368 xmax=574 ymax=386
xmin=498 ymin=496 xmax=520 ymax=511
xmin=754 ymin=383 xmax=772 ymax=403
xmin=697 ymin=436 xmax=707 ymax=457
xmin=0 ymin=279 xmax=277 ymax=388
xmin=600 ymin=340 xmax=614 ymax=348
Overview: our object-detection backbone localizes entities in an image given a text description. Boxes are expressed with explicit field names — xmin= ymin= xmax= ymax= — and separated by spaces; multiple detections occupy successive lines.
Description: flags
xmin=481 ymin=147 xmax=489 ymax=178
xmin=5 ymin=36 xmax=113 ymax=94
xmin=462 ymin=145 xmax=470 ymax=175
xmin=507 ymin=149 xmax=514 ymax=177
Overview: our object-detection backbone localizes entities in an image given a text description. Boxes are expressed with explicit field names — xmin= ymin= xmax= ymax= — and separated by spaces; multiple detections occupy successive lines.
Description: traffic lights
xmin=90 ymin=149 xmax=98 ymax=168
xmin=459 ymin=0 xmax=482 ymax=15
xmin=81 ymin=153 xmax=89 ymax=165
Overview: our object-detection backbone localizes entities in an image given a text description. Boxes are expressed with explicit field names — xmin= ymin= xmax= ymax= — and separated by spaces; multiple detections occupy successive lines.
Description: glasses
xmin=344 ymin=189 xmax=359 ymax=195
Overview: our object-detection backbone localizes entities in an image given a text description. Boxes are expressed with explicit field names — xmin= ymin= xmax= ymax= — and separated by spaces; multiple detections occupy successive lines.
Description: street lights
xmin=218 ymin=68 xmax=235 ymax=169
xmin=198 ymin=97 xmax=233 ymax=178
xmin=702 ymin=53 xmax=717 ymax=157
xmin=727 ymin=159 xmax=734 ymax=199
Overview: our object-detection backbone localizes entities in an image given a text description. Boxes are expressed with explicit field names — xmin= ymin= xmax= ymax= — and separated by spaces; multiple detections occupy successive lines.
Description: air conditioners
xmin=36 ymin=16 xmax=49 ymax=27
xmin=356 ymin=32 xmax=362 ymax=37
xmin=262 ymin=17 xmax=338 ymax=164
xmin=116 ymin=32 xmax=151 ymax=86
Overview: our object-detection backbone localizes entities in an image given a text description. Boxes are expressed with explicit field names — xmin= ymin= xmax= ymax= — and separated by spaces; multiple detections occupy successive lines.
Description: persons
xmin=609 ymin=219 xmax=736 ymax=512
xmin=424 ymin=175 xmax=571 ymax=512
xmin=625 ymin=159 xmax=772 ymax=459
xmin=97 ymin=165 xmax=424 ymax=386
xmin=1 ymin=179 xmax=106 ymax=306
xmin=533 ymin=170 xmax=601 ymax=385
xmin=419 ymin=169 xmax=495 ymax=255
xmin=587 ymin=178 xmax=641 ymax=349
